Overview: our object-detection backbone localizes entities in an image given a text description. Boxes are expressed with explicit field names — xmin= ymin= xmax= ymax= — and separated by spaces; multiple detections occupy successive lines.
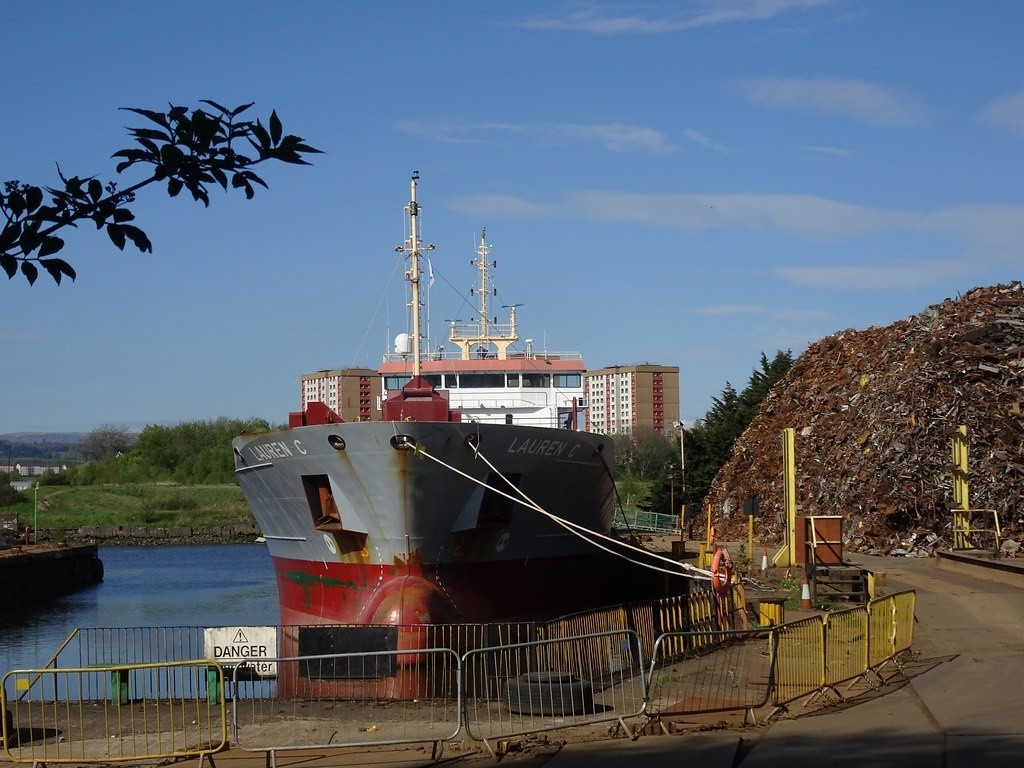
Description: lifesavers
xmin=712 ymin=551 xmax=732 ymax=595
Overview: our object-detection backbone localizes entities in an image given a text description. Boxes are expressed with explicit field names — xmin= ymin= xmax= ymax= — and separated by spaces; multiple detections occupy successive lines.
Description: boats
xmin=233 ymin=170 xmax=671 ymax=698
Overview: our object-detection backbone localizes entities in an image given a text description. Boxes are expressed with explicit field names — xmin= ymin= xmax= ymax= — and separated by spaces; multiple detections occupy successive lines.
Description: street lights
xmin=674 ymin=420 xmax=687 ymax=530
